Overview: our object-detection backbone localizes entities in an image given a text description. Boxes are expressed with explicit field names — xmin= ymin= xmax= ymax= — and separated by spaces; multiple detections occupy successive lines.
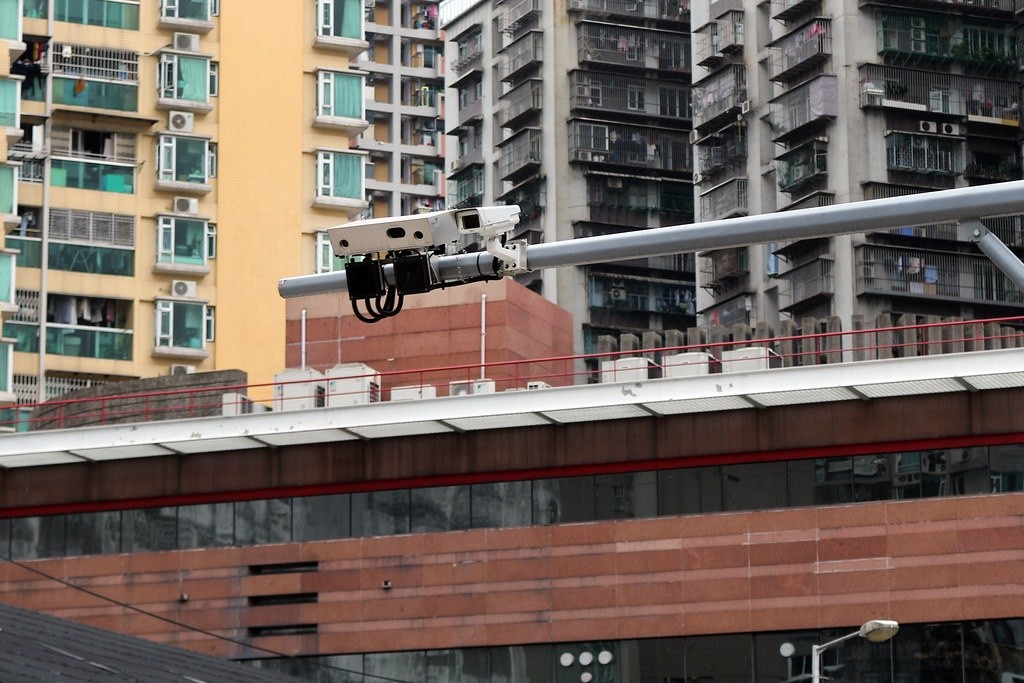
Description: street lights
xmin=812 ymin=619 xmax=900 ymax=683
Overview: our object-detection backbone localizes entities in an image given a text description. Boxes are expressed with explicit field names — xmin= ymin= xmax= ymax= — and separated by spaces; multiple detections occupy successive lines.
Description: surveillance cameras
xmin=449 ymin=203 xmax=521 ymax=238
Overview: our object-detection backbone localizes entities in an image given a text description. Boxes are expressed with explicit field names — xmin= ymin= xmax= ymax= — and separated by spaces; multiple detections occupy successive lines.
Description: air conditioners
xmin=941 ymin=122 xmax=961 ymax=137
xmin=172 ymin=32 xmax=200 ymax=53
xmin=167 ymin=110 xmax=194 ymax=134
xmin=689 ymin=129 xmax=703 ymax=145
xmin=610 ymin=288 xmax=627 ymax=301
xmin=918 ymin=120 xmax=938 ymax=134
xmin=577 ymin=150 xmax=592 ymax=160
xmin=170 ymin=364 xmax=197 ymax=378
xmin=170 ymin=279 xmax=198 ymax=299
xmin=691 ymin=170 xmax=706 ymax=186
xmin=792 ymin=163 xmax=806 ymax=181
xmin=857 ymin=264 xmax=875 ymax=279
xmin=172 ymin=196 xmax=199 ymax=216
xmin=740 ymin=99 xmax=752 ymax=116
xmin=912 ymin=136 xmax=928 ymax=150
xmin=607 ymin=178 xmax=622 ymax=188
xmin=857 ymin=248 xmax=875 ymax=263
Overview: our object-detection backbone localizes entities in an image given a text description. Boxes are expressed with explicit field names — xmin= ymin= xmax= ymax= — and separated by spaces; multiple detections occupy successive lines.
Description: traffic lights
xmin=392 ymin=254 xmax=433 ymax=296
xmin=343 ymin=261 xmax=384 ymax=301
